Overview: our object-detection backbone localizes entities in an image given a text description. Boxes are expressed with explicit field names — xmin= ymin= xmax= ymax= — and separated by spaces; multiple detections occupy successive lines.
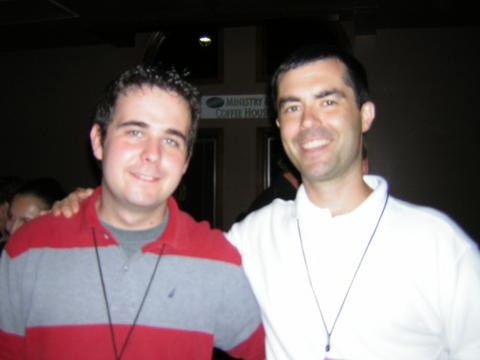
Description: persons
xmin=0 ymin=175 xmax=57 ymax=254
xmin=39 ymin=45 xmax=480 ymax=359
xmin=173 ymin=149 xmax=368 ymax=223
xmin=0 ymin=64 xmax=266 ymax=360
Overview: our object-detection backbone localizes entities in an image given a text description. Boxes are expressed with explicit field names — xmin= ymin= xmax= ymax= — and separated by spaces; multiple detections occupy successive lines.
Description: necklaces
xmin=295 ymin=191 xmax=389 ymax=353
xmin=91 ymin=228 xmax=166 ymax=360
